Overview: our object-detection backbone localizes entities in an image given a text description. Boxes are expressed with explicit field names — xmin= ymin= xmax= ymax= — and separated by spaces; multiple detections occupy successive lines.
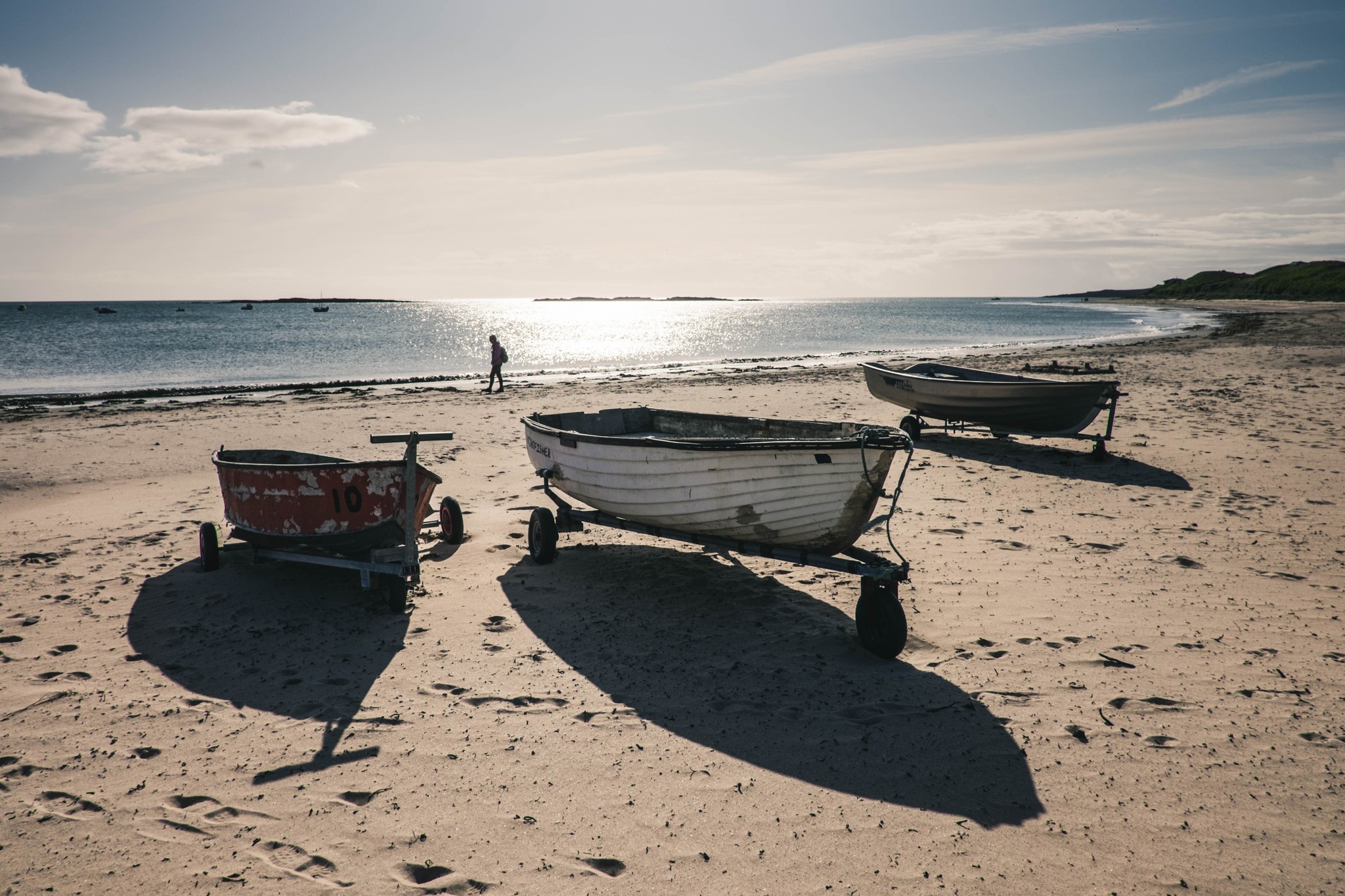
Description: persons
xmin=482 ymin=335 xmax=504 ymax=393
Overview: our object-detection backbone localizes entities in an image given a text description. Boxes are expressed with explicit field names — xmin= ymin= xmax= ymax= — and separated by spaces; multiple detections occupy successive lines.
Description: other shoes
xmin=483 ymin=388 xmax=492 ymax=393
xmin=495 ymin=388 xmax=504 ymax=393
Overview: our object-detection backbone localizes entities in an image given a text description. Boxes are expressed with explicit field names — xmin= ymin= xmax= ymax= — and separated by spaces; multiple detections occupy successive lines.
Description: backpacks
xmin=495 ymin=343 xmax=508 ymax=363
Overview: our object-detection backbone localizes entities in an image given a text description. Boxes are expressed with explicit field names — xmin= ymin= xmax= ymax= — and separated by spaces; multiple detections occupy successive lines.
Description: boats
xmin=212 ymin=446 xmax=443 ymax=556
xmin=519 ymin=406 xmax=915 ymax=555
xmin=856 ymin=359 xmax=1121 ymax=438
